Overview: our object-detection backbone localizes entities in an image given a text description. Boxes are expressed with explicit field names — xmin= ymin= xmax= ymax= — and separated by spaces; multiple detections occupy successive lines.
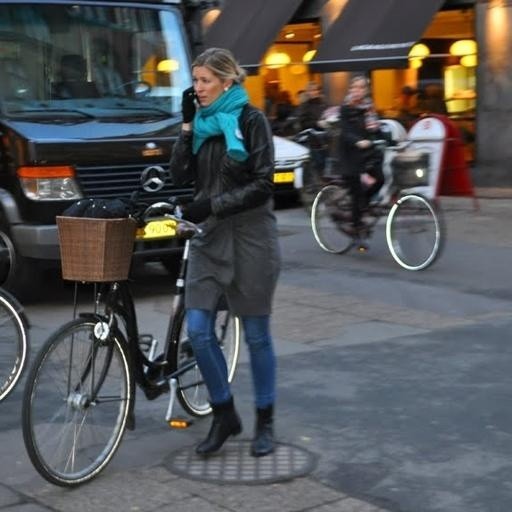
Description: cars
xmin=273 ymin=135 xmax=311 ymax=192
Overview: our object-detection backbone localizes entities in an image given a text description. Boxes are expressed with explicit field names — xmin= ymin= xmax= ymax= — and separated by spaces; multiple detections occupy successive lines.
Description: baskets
xmin=55 ymin=216 xmax=137 ymax=282
xmin=391 ymin=152 xmax=431 ymax=188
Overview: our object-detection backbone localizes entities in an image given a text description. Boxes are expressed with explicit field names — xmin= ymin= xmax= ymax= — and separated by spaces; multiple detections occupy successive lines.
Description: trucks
xmin=0 ymin=1 xmax=198 ymax=300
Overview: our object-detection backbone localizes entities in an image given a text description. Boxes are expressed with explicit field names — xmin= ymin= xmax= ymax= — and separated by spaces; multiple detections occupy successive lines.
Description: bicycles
xmin=0 ymin=287 xmax=33 ymax=403
xmin=19 ymin=201 xmax=241 ymax=489
xmin=311 ymin=132 xmax=445 ymax=272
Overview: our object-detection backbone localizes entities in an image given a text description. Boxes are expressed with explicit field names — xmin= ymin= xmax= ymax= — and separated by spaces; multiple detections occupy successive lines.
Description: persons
xmin=170 ymin=47 xmax=281 ymax=456
xmin=265 ymin=73 xmax=447 ymax=235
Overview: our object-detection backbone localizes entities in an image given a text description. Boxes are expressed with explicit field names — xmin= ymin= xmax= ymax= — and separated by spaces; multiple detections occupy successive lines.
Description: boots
xmin=249 ymin=400 xmax=276 ymax=457
xmin=196 ymin=395 xmax=243 ymax=457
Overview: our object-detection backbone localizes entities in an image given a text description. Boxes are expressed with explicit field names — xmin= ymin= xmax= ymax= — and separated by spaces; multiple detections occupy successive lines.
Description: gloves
xmin=181 ymin=198 xmax=213 ymax=225
xmin=182 ymin=85 xmax=196 ymax=123
